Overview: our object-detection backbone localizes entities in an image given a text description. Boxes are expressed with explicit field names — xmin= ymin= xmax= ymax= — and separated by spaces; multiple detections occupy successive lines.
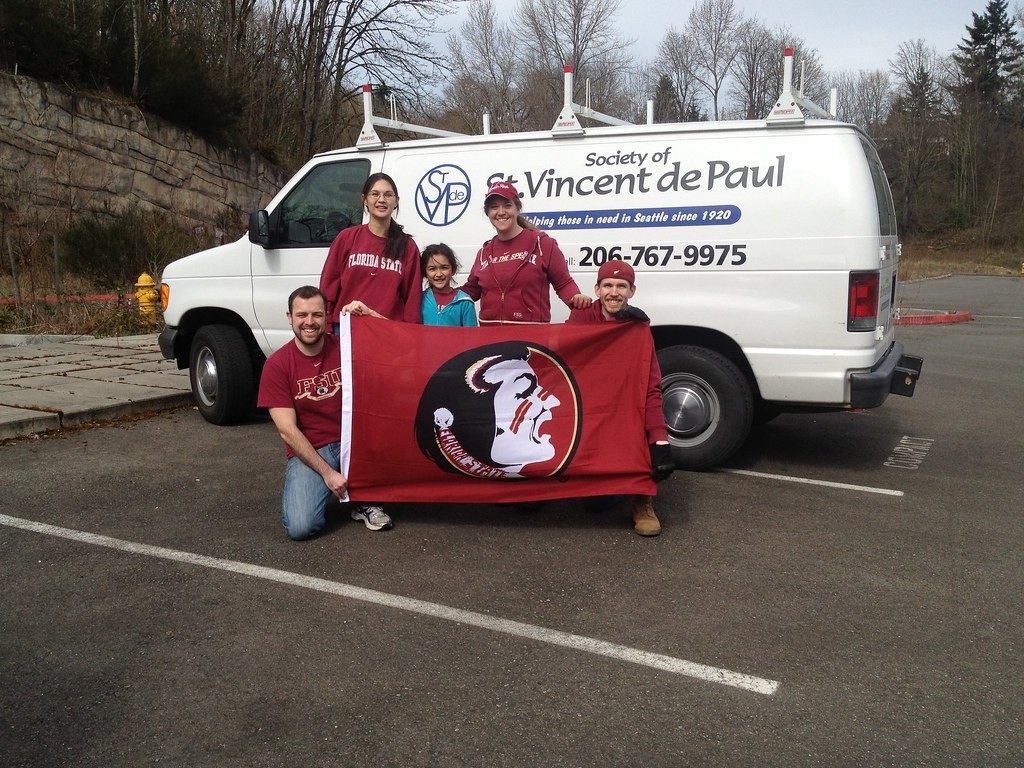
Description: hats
xmin=597 ymin=261 xmax=636 ymax=287
xmin=484 ymin=181 xmax=519 ymax=204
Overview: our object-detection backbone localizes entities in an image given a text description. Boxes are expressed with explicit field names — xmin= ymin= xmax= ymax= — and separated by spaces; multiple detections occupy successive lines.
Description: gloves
xmin=614 ymin=305 xmax=651 ymax=322
xmin=648 ymin=444 xmax=676 ymax=485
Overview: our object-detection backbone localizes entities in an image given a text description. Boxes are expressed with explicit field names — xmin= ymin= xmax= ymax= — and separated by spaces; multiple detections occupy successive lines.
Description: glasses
xmin=366 ymin=192 xmax=398 ymax=200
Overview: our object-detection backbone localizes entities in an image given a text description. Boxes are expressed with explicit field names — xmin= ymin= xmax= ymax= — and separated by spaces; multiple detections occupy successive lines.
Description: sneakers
xmin=622 ymin=494 xmax=663 ymax=537
xmin=351 ymin=502 xmax=394 ymax=531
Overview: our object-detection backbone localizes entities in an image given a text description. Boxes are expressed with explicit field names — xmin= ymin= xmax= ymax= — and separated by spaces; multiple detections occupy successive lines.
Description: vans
xmin=157 ymin=48 xmax=922 ymax=466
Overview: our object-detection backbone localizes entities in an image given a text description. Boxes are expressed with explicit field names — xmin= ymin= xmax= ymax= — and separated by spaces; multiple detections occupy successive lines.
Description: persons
xmin=257 ymin=286 xmax=394 ymax=541
xmin=566 ymin=261 xmax=678 ymax=537
xmin=419 ymin=243 xmax=478 ymax=327
xmin=318 ymin=173 xmax=422 ymax=335
xmin=452 ymin=181 xmax=592 ymax=507
